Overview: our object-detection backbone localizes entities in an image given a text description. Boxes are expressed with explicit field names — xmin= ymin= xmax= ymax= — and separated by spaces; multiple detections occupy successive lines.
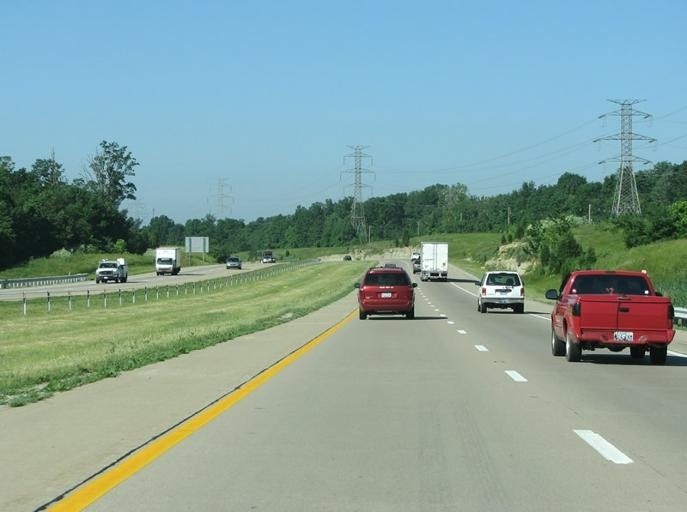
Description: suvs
xmin=95 ymin=258 xmax=128 ymax=283
xmin=474 ymin=270 xmax=524 ymax=313
xmin=410 ymin=252 xmax=420 ymax=261
xmin=343 ymin=256 xmax=351 ymax=261
xmin=413 ymin=260 xmax=420 ymax=274
xmin=354 ymin=264 xmax=417 ymax=320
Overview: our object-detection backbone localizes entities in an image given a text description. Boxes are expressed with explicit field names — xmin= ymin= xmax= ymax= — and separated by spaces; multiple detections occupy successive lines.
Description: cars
xmin=225 ymin=257 xmax=242 ymax=269
xmin=263 ymin=256 xmax=272 ymax=263
xmin=271 ymin=258 xmax=276 ymax=263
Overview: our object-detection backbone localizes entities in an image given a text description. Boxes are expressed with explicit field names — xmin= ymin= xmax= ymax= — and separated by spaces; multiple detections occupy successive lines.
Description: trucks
xmin=420 ymin=242 xmax=448 ymax=282
xmin=155 ymin=246 xmax=181 ymax=275
xmin=256 ymin=251 xmax=272 ymax=263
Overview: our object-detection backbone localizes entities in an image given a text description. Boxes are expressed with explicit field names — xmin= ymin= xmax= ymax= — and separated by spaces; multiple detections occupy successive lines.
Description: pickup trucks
xmin=544 ymin=270 xmax=676 ymax=364
